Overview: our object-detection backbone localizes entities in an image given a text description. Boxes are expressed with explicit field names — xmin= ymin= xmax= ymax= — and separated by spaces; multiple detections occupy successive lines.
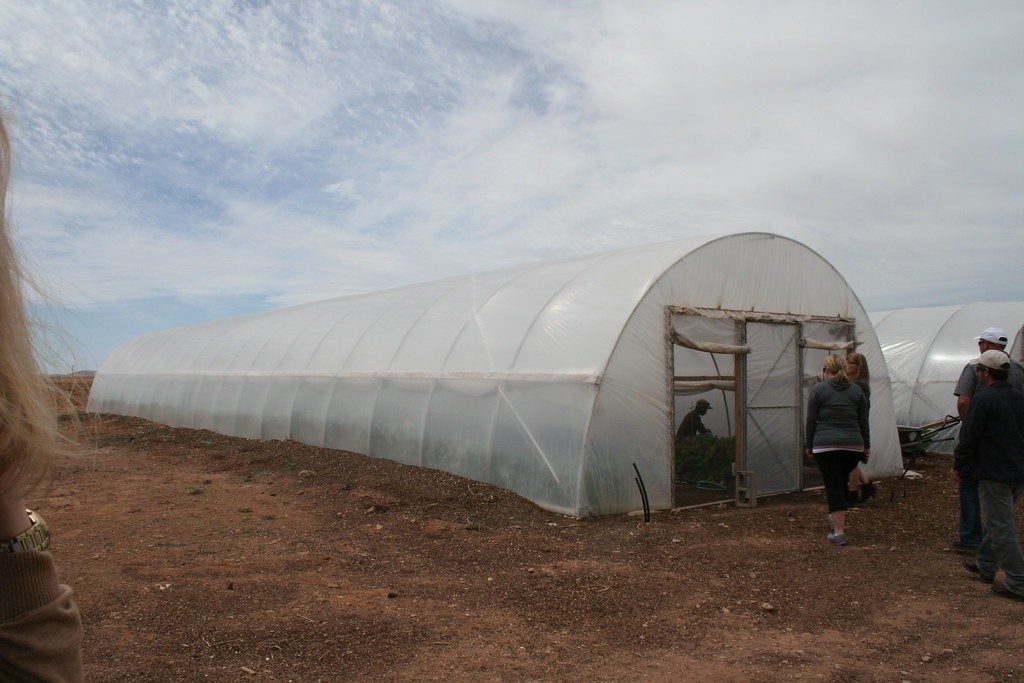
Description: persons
xmin=677 ymin=399 xmax=714 ymax=438
xmin=844 ymin=352 xmax=877 ymax=505
xmin=805 ymin=354 xmax=871 ymax=546
xmin=952 ymin=326 xmax=1024 ymax=547
xmin=0 ymin=116 xmax=84 ymax=683
xmin=950 ymin=350 xmax=1024 ymax=601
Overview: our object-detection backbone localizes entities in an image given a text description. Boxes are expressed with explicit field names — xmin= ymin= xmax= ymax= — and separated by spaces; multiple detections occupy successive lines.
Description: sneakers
xmin=991 ymin=577 xmax=1024 ymax=600
xmin=828 ymin=514 xmax=835 ymax=530
xmin=960 ymin=562 xmax=994 ymax=584
xmin=827 ymin=533 xmax=848 ymax=546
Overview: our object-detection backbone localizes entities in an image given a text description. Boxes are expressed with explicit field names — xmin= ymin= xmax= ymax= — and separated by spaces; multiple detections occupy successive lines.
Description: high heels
xmin=859 ymin=480 xmax=877 ymax=503
xmin=849 ymin=491 xmax=859 ymax=506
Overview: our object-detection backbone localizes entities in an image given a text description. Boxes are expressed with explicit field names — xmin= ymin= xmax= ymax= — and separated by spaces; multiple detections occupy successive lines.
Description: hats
xmin=697 ymin=399 xmax=713 ymax=409
xmin=973 ymin=327 xmax=1009 ymax=345
xmin=969 ymin=350 xmax=1011 ymax=371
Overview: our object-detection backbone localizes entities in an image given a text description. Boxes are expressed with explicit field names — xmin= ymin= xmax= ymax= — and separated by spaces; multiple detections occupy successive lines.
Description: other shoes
xmin=953 ymin=546 xmax=978 ymax=557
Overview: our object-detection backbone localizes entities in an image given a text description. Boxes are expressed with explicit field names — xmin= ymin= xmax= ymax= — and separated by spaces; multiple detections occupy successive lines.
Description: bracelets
xmin=0 ymin=508 xmax=51 ymax=553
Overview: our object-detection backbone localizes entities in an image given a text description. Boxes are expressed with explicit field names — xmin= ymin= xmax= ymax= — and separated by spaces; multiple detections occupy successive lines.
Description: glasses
xmin=823 ymin=368 xmax=830 ymax=373
